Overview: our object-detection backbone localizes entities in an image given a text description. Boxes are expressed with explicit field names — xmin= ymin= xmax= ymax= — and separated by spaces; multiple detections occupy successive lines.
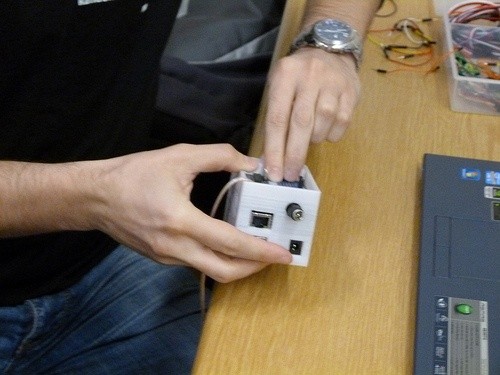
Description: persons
xmin=0 ymin=0 xmax=384 ymax=375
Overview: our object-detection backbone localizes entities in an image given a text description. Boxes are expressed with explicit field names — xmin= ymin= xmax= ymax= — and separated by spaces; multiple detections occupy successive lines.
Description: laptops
xmin=413 ymin=154 xmax=500 ymax=375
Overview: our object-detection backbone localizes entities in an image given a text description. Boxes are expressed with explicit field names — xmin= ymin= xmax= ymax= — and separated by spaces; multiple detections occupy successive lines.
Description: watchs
xmin=287 ymin=18 xmax=363 ymax=72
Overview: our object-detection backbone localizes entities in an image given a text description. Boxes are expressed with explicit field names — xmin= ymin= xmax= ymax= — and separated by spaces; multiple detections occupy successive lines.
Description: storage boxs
xmin=442 ymin=11 xmax=500 ymax=115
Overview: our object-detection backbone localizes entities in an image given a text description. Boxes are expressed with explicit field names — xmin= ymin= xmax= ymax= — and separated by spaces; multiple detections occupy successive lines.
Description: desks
xmin=191 ymin=0 xmax=500 ymax=375
